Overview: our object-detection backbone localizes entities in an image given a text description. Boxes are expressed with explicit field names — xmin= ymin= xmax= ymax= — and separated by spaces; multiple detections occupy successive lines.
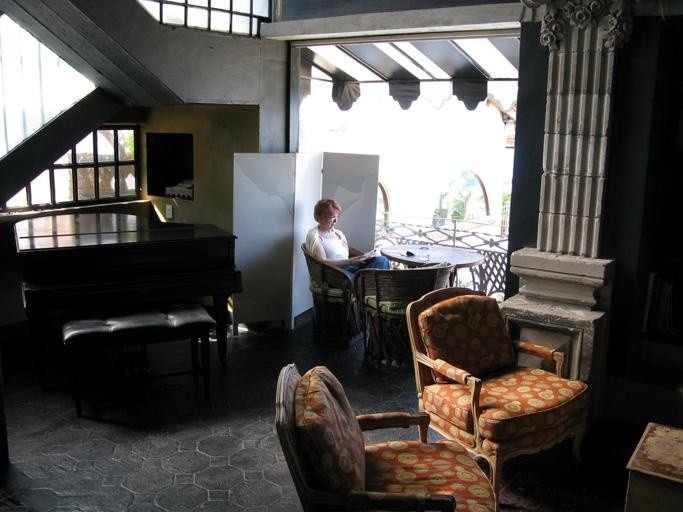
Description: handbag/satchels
xmin=367 ymin=325 xmax=398 ymax=361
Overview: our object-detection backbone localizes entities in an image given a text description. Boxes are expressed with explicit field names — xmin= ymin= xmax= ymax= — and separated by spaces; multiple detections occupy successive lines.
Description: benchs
xmin=61 ymin=302 xmax=216 ymax=417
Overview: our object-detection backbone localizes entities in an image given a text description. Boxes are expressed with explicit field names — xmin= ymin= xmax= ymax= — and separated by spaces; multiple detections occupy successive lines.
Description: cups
xmin=418 ymin=247 xmax=430 ymax=261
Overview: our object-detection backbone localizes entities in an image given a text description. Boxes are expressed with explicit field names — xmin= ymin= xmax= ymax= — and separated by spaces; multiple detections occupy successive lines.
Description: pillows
xmin=418 ymin=295 xmax=516 ymax=384
xmin=295 ymin=365 xmax=366 ymax=494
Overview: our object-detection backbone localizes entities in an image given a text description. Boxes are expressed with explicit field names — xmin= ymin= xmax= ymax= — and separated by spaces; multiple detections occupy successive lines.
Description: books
xmin=360 ymin=247 xmax=381 ymax=261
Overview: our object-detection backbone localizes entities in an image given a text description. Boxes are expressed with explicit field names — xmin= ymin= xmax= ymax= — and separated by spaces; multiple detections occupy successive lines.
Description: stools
xmin=624 ymin=422 xmax=683 ymax=512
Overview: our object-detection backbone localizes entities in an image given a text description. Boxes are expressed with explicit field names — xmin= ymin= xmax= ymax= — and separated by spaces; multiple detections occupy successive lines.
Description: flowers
xmin=540 ymin=0 xmax=633 ymax=50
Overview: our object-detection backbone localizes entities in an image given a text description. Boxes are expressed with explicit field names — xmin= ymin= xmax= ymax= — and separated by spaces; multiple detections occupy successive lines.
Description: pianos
xmin=13 ymin=201 xmax=242 ymax=362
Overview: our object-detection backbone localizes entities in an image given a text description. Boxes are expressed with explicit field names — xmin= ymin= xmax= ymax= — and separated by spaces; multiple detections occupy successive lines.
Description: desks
xmin=380 ymin=245 xmax=485 ymax=287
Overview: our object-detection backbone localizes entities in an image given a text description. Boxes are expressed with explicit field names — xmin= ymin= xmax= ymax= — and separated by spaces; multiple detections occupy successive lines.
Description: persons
xmin=306 ymin=198 xmax=389 ymax=289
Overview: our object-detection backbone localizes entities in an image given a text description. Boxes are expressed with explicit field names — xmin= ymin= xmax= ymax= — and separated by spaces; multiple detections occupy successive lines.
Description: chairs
xmin=301 ymin=242 xmax=365 ymax=348
xmin=406 ymin=288 xmax=592 ymax=503
xmin=276 ymin=362 xmax=497 ymax=512
xmin=354 ymin=262 xmax=457 ymax=368
